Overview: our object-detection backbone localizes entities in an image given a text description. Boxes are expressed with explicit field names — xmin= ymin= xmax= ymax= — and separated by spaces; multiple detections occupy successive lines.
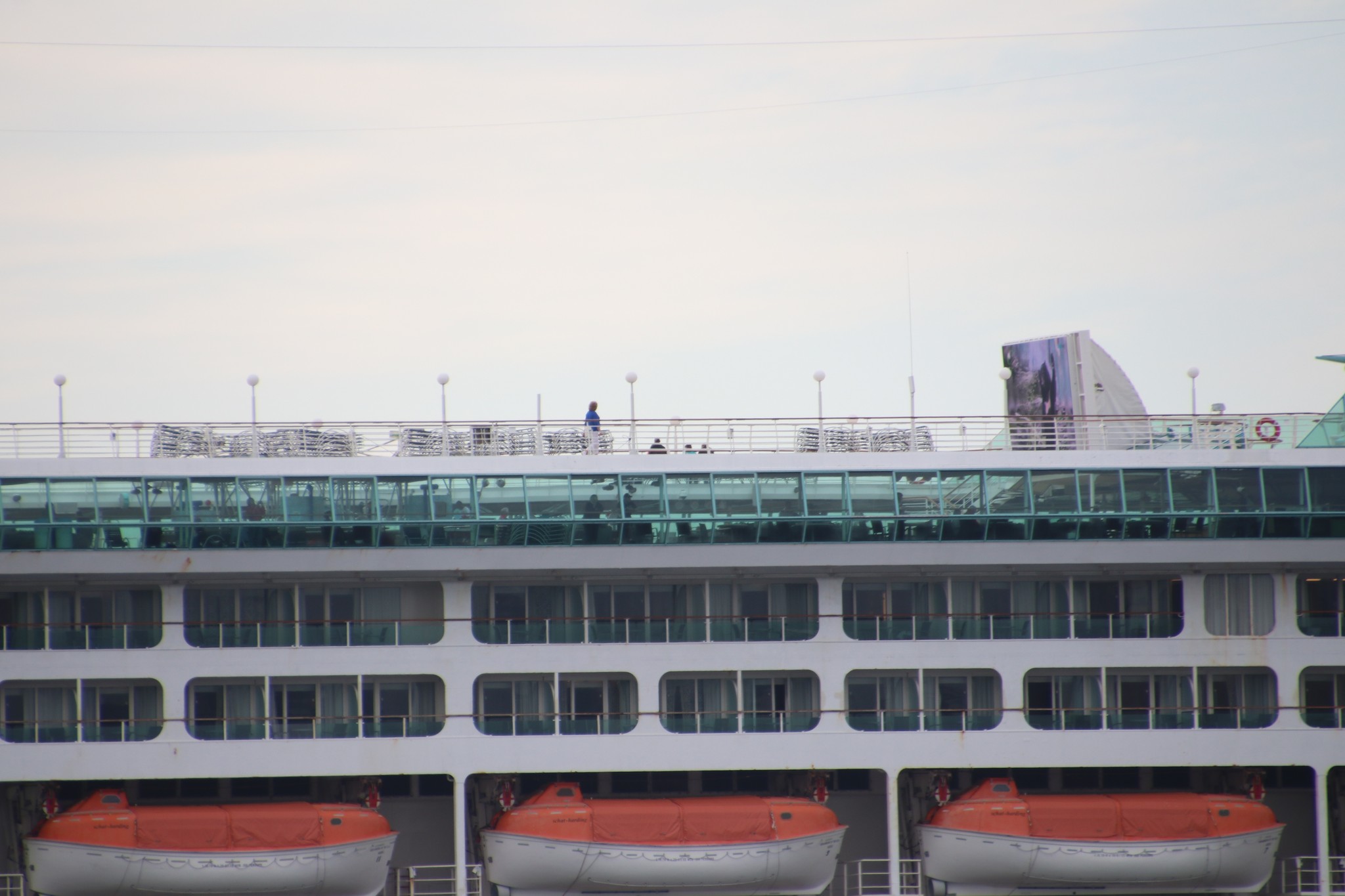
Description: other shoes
xmin=581 ymin=450 xmax=589 ymax=456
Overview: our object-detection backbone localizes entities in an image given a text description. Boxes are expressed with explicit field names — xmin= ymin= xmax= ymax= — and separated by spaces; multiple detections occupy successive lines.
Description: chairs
xmin=3 ymin=508 xmax=1344 ymax=550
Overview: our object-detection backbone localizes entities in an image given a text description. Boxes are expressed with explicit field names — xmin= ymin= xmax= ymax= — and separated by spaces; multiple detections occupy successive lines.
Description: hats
xmin=623 ymin=493 xmax=632 ymax=498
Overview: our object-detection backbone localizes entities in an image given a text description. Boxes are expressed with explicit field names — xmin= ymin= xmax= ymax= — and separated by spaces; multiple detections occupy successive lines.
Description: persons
xmin=681 ymin=444 xmax=697 ymax=454
xmin=893 ymin=492 xmax=906 ymax=541
xmin=580 ymin=401 xmax=602 ymax=455
xmin=453 ymin=501 xmax=471 ymax=520
xmin=616 ymin=493 xmax=637 ymax=532
xmin=582 ymin=494 xmax=603 ymax=544
xmin=698 ymin=444 xmax=714 ymax=454
xmin=244 ymin=498 xmax=266 ymax=522
xmin=647 ymin=438 xmax=667 ymax=454
xmin=498 ymin=508 xmax=509 ymax=520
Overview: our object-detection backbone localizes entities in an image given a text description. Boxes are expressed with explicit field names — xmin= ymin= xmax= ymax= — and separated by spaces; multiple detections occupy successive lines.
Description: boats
xmin=0 ymin=330 xmax=1345 ymax=896
xmin=914 ymin=777 xmax=1287 ymax=896
xmin=480 ymin=781 xmax=850 ymax=896
xmin=23 ymin=789 xmax=400 ymax=896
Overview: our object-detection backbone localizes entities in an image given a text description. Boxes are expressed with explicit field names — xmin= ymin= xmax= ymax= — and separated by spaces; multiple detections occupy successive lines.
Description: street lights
xmin=813 ymin=369 xmax=827 ymax=452
xmin=52 ymin=375 xmax=66 ymax=461
xmin=130 ymin=420 xmax=143 ymax=457
xmin=999 ymin=366 xmax=1013 ymax=450
xmin=623 ymin=372 xmax=638 ymax=455
xmin=1186 ymin=366 xmax=1200 ymax=450
xmin=246 ymin=372 xmax=260 ymax=456
xmin=436 ymin=373 xmax=450 ymax=455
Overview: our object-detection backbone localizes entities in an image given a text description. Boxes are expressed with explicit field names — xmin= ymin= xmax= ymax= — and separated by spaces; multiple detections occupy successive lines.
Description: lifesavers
xmin=1256 ymin=416 xmax=1281 ymax=441
